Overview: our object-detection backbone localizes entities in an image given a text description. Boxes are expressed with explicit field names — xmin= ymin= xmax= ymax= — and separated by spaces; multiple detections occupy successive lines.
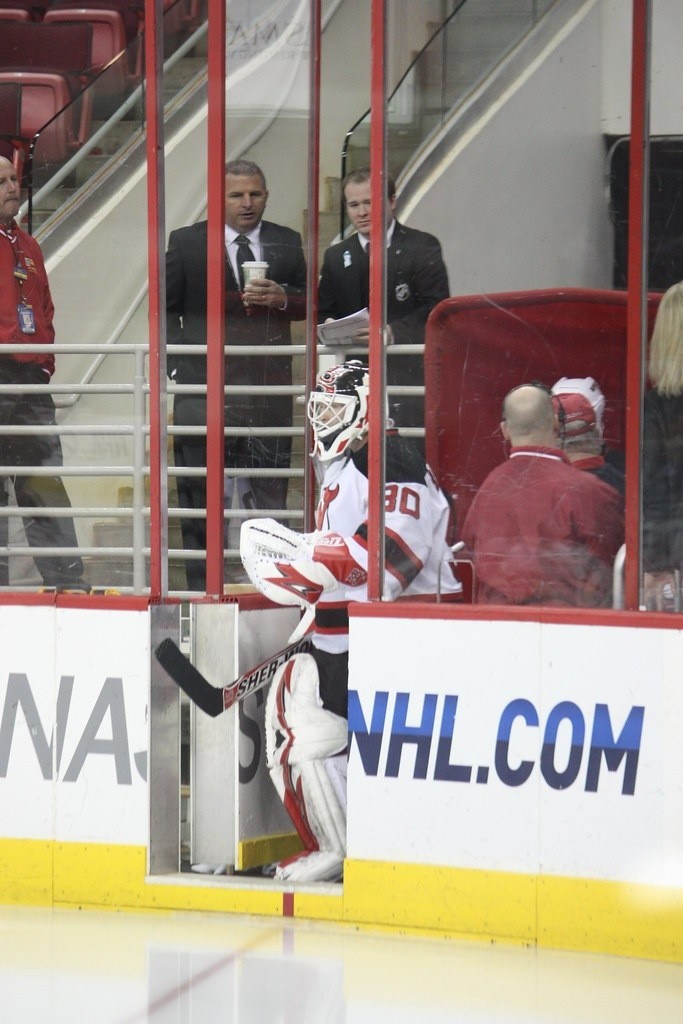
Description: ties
xmin=231 ymin=235 xmax=256 ymax=294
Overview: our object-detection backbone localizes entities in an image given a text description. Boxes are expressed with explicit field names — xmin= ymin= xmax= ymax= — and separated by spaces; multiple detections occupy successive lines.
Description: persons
xmin=456 ymin=383 xmax=626 ymax=611
xmin=549 ymin=376 xmax=627 ymax=497
xmin=164 ymin=158 xmax=309 ymax=592
xmin=0 ymin=154 xmax=91 ymax=587
xmin=641 ymin=281 xmax=683 ymax=613
xmin=316 ymin=166 xmax=452 ymax=460
xmin=235 ymin=359 xmax=466 ymax=884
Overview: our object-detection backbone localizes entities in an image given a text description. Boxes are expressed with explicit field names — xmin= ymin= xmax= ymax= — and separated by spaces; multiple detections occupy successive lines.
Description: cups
xmin=242 ymin=260 xmax=269 ymax=299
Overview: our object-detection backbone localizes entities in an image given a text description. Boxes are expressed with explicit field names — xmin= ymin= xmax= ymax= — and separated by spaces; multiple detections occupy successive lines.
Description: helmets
xmin=309 ymin=361 xmax=396 ymax=460
xmin=549 ymin=376 xmax=609 ymax=435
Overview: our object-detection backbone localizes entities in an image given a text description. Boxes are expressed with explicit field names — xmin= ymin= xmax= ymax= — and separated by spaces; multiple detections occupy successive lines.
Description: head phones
xmin=500 ymin=380 xmax=566 ymax=423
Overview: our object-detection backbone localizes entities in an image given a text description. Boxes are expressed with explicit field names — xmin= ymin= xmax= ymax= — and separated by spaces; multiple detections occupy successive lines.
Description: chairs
xmin=0 ymin=1 xmax=202 ymax=189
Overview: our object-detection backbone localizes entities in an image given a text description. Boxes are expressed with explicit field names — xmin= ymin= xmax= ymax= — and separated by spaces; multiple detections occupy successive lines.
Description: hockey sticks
xmin=153 ymin=633 xmax=313 ymax=718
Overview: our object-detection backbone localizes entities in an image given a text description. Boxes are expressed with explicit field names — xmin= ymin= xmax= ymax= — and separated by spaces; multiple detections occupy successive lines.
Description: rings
xmin=263 ymin=295 xmax=266 ymax=301
